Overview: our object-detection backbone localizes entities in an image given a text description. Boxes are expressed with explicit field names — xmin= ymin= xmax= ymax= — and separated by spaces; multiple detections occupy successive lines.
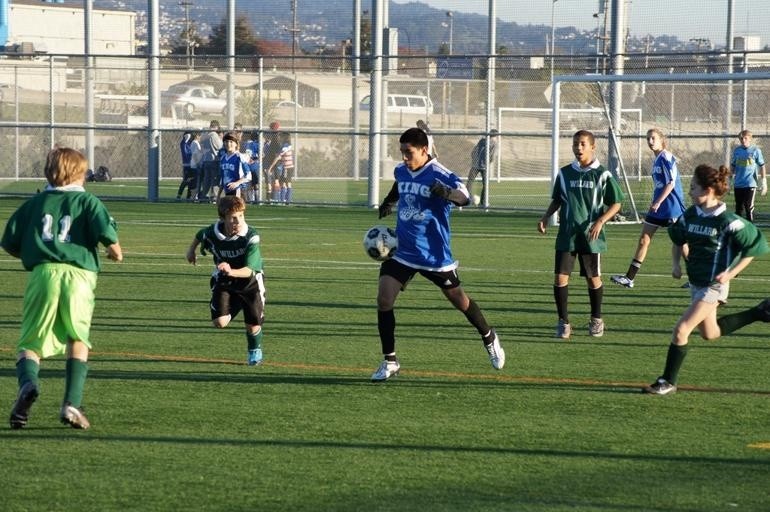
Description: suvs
xmin=544 ymin=101 xmax=627 ymax=138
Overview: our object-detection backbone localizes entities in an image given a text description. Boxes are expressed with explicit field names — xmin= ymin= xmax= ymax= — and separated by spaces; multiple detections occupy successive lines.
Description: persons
xmin=2 ymin=147 xmax=122 ymax=430
xmin=416 ymin=120 xmax=437 ymax=158
xmin=465 ymin=128 xmax=499 ymax=208
xmin=176 ymin=120 xmax=295 ymax=207
xmin=185 ymin=198 xmax=266 ymax=365
xmin=725 ymin=130 xmax=768 ymax=223
xmin=641 ymin=164 xmax=770 ymax=395
xmin=537 ymin=131 xmax=624 ymax=340
xmin=609 ymin=126 xmax=690 ymax=289
xmin=369 ymin=126 xmax=505 ymax=382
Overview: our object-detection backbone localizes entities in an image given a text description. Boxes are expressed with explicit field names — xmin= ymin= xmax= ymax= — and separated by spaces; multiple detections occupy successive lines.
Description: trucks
xmin=67 ymin=54 xmax=162 ymax=97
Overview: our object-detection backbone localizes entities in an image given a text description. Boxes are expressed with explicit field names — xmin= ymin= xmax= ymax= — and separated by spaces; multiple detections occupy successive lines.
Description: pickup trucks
xmin=66 ymin=68 xmax=85 ymax=90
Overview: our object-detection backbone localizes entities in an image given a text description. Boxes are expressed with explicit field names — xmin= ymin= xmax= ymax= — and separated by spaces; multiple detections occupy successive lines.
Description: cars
xmin=0 ymin=83 xmax=33 ymax=89
xmin=468 ymin=100 xmax=487 ymax=116
xmin=1 ymin=41 xmax=48 ymax=62
xmin=710 ymin=95 xmax=743 ymax=119
xmin=37 ymin=53 xmax=69 ymax=62
xmin=160 ymin=84 xmax=228 ymax=119
xmin=263 ymin=98 xmax=304 ymax=108
xmin=433 ymin=103 xmax=455 ymax=115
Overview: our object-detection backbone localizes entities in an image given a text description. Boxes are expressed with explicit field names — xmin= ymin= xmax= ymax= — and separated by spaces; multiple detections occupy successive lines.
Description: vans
xmin=348 ymin=94 xmax=433 ymax=115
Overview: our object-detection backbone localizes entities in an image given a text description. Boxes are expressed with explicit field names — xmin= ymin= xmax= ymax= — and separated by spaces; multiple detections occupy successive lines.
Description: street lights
xmin=551 ymin=1 xmax=558 ymax=83
xmin=446 ymin=11 xmax=454 ymax=56
xmin=593 ymin=12 xmax=601 ymax=77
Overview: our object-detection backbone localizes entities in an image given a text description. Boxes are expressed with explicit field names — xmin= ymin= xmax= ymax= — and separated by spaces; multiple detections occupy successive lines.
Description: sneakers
xmin=589 ymin=317 xmax=604 ymax=337
xmin=644 ymin=377 xmax=677 ymax=395
xmin=370 ymin=362 xmax=400 ymax=382
xmin=8 ymin=382 xmax=39 ymax=429
xmin=558 ymin=319 xmax=570 ymax=339
xmin=611 ymin=274 xmax=634 ymax=289
xmin=59 ymin=402 xmax=90 ymax=429
xmin=248 ymin=345 xmax=263 ymax=366
xmin=485 ymin=332 xmax=504 ymax=370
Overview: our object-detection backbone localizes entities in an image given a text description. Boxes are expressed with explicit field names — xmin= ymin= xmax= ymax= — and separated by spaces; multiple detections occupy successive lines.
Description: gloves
xmin=378 ymin=202 xmax=391 ymax=218
xmin=432 ymin=179 xmax=451 ymax=199
xmin=759 ymin=178 xmax=767 ymax=196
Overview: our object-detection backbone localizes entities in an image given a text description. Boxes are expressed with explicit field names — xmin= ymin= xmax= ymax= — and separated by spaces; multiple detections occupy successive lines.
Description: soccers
xmin=363 ymin=226 xmax=399 ymax=261
